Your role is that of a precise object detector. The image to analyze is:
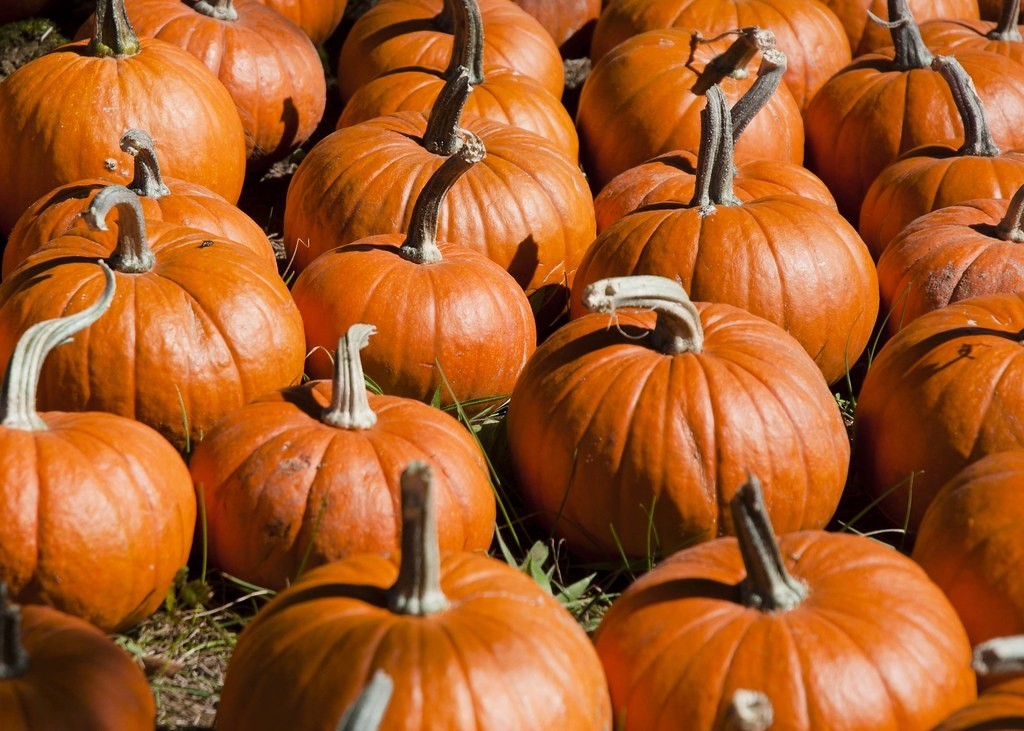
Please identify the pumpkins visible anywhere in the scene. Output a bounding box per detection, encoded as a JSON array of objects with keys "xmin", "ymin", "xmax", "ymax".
[{"xmin": 2, "ymin": 0, "xmax": 1022, "ymax": 731}]
